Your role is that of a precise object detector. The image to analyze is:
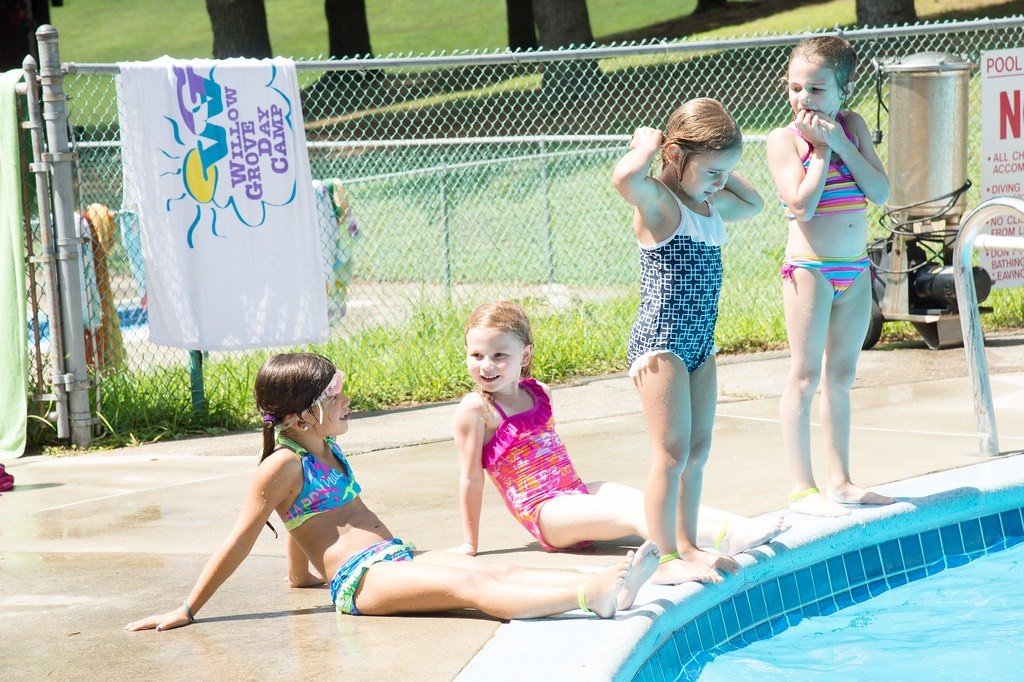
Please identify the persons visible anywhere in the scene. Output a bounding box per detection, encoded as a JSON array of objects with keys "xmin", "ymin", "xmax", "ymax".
[
  {"xmin": 123, "ymin": 351, "xmax": 661, "ymax": 635},
  {"xmin": 761, "ymin": 34, "xmax": 899, "ymax": 516},
  {"xmin": 446, "ymin": 298, "xmax": 803, "ymax": 561},
  {"xmin": 607, "ymin": 94, "xmax": 767, "ymax": 587}
]
